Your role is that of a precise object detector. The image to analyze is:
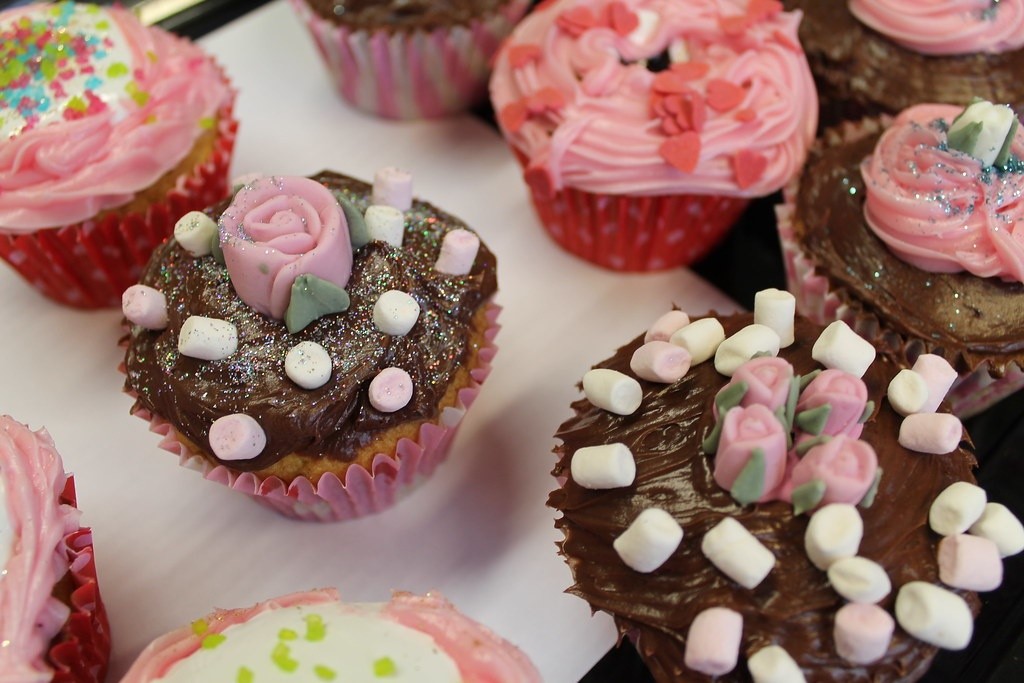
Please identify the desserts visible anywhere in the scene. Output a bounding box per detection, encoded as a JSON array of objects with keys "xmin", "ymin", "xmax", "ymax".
[{"xmin": 0, "ymin": 0, "xmax": 1024, "ymax": 683}]
